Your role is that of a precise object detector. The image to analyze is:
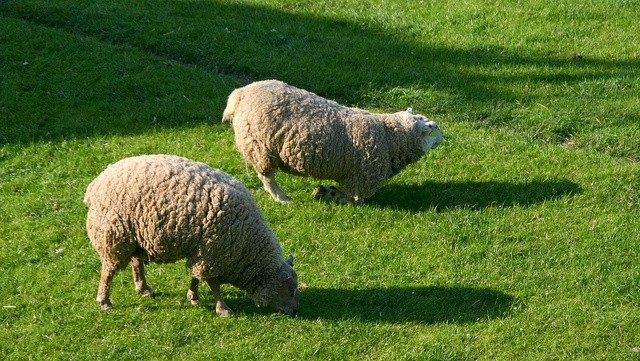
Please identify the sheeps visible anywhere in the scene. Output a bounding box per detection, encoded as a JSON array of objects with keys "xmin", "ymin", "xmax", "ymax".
[
  {"xmin": 83, "ymin": 154, "xmax": 298, "ymax": 319},
  {"xmin": 222, "ymin": 79, "xmax": 443, "ymax": 205}
]
